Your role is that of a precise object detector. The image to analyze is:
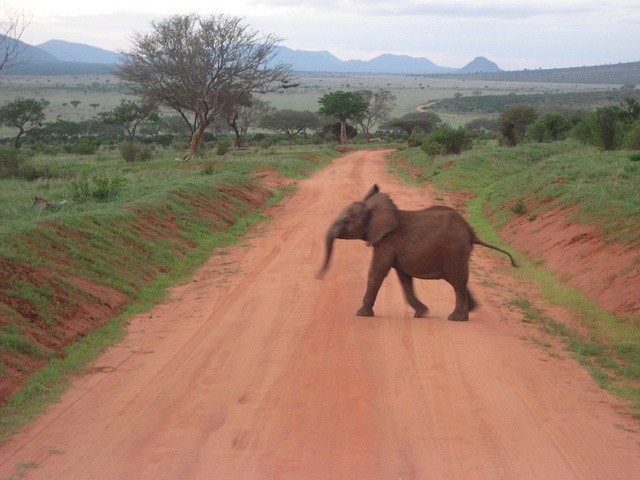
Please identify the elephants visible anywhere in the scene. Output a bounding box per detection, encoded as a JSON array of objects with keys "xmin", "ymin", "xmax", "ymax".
[{"xmin": 314, "ymin": 183, "xmax": 522, "ymax": 321}]
